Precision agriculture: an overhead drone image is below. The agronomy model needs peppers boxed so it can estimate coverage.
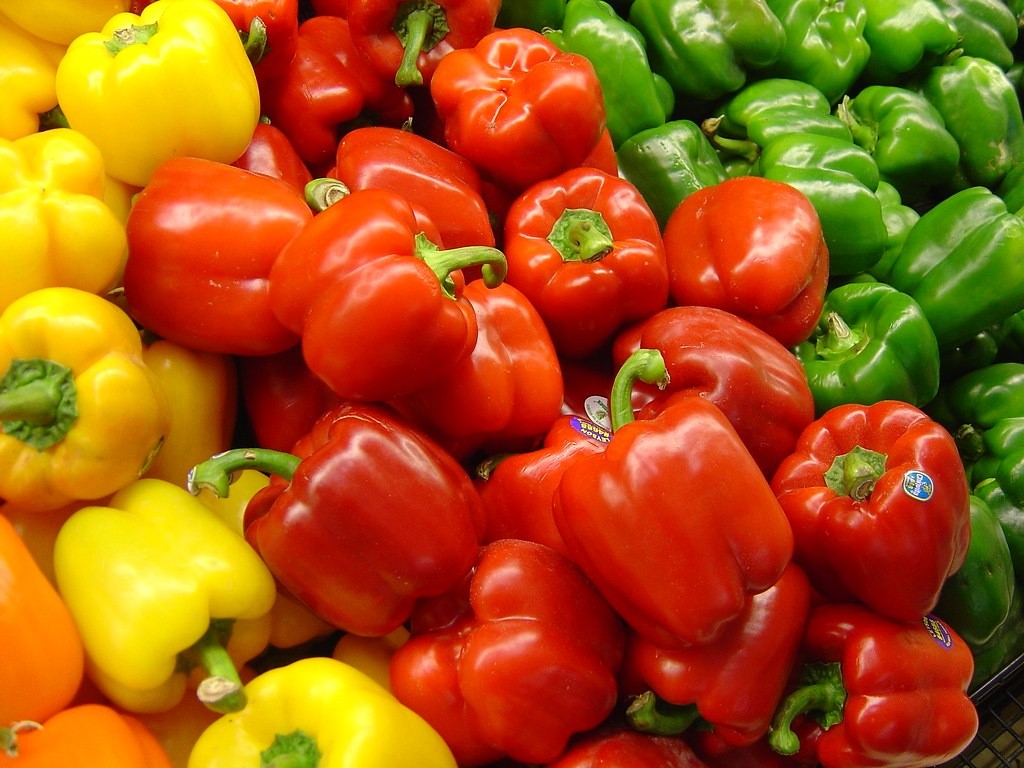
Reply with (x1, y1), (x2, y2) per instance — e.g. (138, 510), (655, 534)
(0, 0), (1024, 768)
